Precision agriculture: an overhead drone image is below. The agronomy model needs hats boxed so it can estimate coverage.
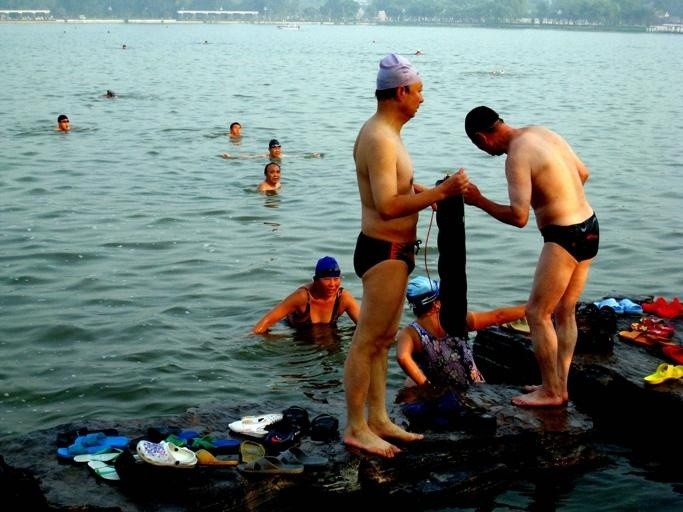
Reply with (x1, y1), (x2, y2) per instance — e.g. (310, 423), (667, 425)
(406, 276), (437, 307)
(376, 53), (422, 91)
(57, 115), (67, 122)
(315, 256), (340, 278)
(269, 139), (279, 148)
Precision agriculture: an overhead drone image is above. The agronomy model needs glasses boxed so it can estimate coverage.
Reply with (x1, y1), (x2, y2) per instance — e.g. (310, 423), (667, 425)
(316, 269), (339, 277)
(271, 145), (280, 148)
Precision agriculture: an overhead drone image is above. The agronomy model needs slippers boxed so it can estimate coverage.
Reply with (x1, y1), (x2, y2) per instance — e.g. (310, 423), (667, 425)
(498, 295), (682, 386)
(56, 404), (342, 481)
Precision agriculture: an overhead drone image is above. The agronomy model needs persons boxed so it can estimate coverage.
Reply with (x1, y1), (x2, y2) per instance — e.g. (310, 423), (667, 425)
(221, 139), (320, 160)
(255, 163), (280, 192)
(224, 122), (241, 140)
(340, 52), (471, 459)
(248, 253), (359, 335)
(54, 114), (70, 131)
(396, 276), (527, 387)
(461, 106), (600, 407)
(102, 90), (119, 99)
